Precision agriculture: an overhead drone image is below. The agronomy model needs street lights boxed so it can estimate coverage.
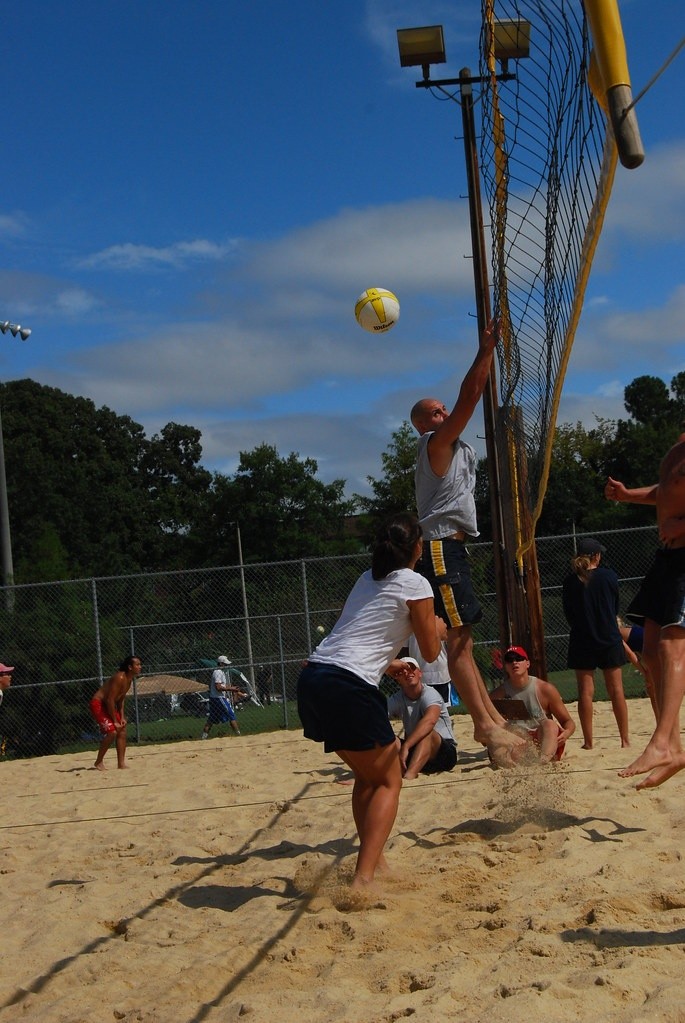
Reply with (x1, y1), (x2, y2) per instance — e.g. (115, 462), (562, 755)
(0, 315), (31, 614)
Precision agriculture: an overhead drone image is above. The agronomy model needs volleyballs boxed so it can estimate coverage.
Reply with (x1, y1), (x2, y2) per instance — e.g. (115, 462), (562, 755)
(354, 286), (400, 334)
(317, 625), (325, 634)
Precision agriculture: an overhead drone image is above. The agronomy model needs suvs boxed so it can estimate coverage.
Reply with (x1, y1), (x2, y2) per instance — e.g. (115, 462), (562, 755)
(132, 696), (156, 711)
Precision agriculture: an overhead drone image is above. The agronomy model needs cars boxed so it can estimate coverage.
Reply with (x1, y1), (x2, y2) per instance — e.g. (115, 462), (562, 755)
(165, 691), (231, 714)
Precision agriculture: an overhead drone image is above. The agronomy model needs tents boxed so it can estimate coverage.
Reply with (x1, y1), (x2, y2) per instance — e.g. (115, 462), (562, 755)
(126, 675), (210, 700)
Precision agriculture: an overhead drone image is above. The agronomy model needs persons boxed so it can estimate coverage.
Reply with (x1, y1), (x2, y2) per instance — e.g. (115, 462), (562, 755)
(87, 656), (142, 771)
(296, 517), (448, 894)
(489, 643), (503, 688)
(606, 419), (685, 793)
(563, 539), (657, 749)
(487, 647), (575, 769)
(410, 320), (527, 747)
(256, 666), (272, 705)
(338, 657), (457, 784)
(199, 656), (248, 739)
(0, 663), (14, 705)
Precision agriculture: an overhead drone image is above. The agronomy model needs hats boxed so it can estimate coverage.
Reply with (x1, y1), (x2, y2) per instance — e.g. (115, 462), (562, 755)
(0, 662), (15, 674)
(503, 645), (532, 663)
(215, 656), (231, 665)
(397, 657), (422, 671)
(577, 538), (608, 560)
